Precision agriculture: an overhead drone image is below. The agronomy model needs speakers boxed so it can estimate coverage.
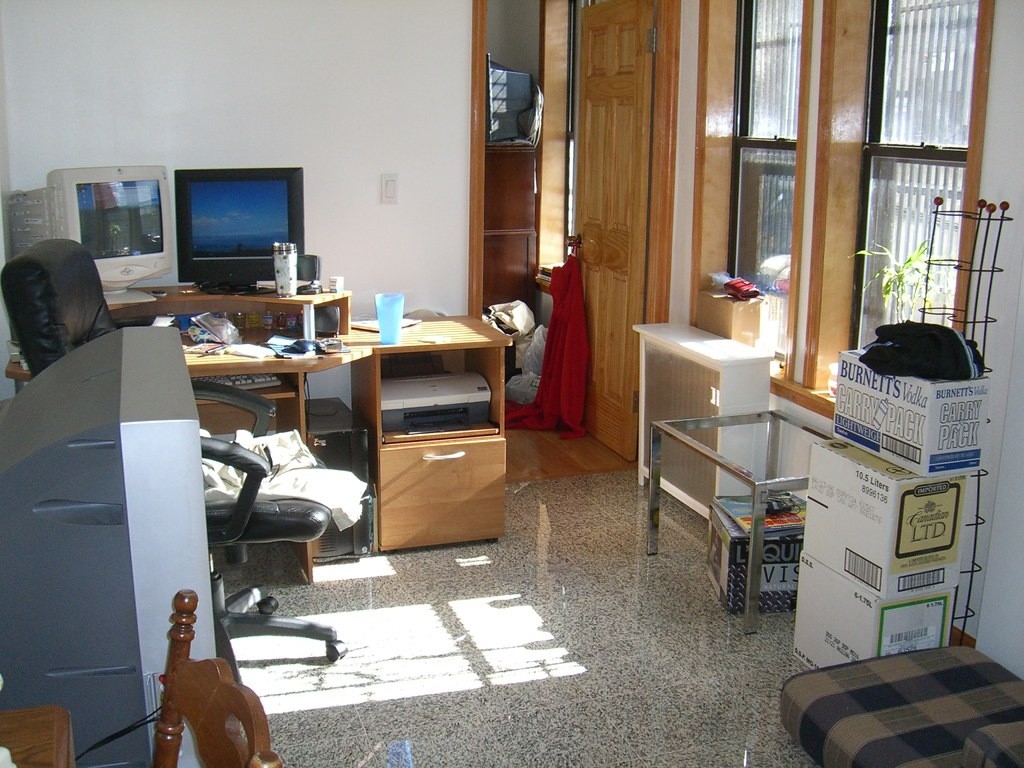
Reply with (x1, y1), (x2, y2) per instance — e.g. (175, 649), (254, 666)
(314, 305), (340, 337)
(296, 253), (322, 296)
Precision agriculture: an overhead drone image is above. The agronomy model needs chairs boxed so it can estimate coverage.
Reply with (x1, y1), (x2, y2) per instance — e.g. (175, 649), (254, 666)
(152, 587), (289, 768)
(0, 236), (351, 683)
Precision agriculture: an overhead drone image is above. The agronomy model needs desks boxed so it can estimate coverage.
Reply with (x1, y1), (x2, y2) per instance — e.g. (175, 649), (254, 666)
(0, 704), (75, 768)
(6, 285), (364, 564)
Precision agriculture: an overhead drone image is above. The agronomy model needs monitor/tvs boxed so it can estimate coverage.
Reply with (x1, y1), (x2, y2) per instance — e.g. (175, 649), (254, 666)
(173, 167), (304, 294)
(485, 52), (534, 144)
(7, 165), (174, 305)
(0, 325), (216, 768)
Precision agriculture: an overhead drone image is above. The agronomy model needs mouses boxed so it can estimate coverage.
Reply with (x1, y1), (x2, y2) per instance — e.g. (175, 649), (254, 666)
(289, 339), (314, 353)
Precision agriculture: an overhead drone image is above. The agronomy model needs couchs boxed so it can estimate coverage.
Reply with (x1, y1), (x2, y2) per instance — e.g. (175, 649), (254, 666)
(779, 645), (1024, 768)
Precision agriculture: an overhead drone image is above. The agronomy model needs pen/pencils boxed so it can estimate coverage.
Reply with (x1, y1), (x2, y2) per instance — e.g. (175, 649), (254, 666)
(205, 345), (227, 354)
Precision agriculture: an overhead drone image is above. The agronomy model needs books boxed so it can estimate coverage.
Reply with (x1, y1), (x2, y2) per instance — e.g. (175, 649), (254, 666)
(536, 262), (564, 286)
(713, 493), (807, 535)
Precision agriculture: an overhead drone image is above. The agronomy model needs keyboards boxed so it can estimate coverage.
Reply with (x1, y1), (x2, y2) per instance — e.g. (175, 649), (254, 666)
(192, 372), (281, 390)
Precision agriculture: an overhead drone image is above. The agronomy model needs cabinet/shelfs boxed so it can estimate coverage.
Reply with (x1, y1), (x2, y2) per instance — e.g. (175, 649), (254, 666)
(484, 137), (536, 320)
(353, 309), (516, 555)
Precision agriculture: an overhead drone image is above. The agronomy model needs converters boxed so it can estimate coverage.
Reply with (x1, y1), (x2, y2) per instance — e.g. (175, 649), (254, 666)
(768, 500), (795, 513)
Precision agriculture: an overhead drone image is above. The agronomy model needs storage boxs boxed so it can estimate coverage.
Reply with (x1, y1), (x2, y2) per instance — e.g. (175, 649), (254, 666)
(792, 553), (956, 673)
(697, 287), (766, 336)
(803, 438), (970, 600)
(702, 493), (808, 614)
(832, 345), (995, 478)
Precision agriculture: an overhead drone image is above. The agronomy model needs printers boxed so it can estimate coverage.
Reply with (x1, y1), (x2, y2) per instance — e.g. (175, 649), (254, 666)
(380, 371), (492, 434)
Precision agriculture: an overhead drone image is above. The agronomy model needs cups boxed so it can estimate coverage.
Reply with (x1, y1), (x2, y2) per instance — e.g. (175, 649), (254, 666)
(376, 293), (405, 345)
(274, 243), (298, 298)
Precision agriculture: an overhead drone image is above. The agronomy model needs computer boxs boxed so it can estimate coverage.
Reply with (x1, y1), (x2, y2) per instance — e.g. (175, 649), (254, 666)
(304, 397), (371, 563)
